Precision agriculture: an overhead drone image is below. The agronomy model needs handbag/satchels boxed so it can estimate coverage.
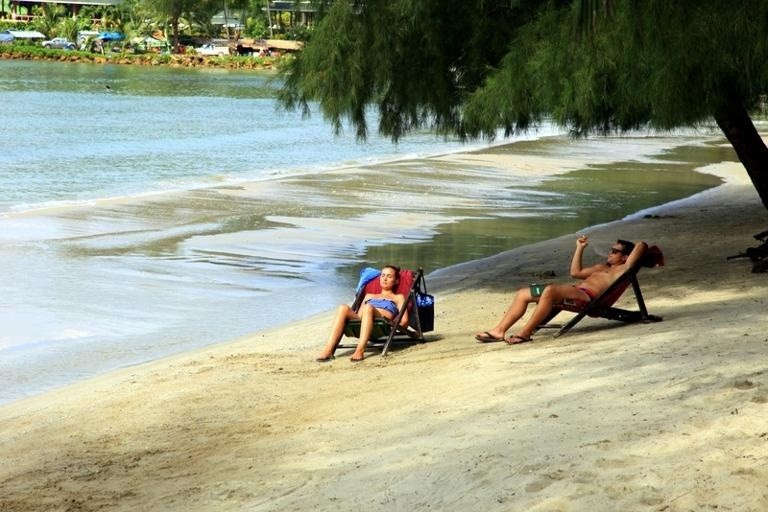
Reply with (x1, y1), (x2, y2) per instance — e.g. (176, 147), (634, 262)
(409, 291), (434, 332)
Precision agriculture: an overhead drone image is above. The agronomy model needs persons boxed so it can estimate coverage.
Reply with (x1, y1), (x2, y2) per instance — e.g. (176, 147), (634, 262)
(473, 233), (650, 344)
(316, 264), (409, 363)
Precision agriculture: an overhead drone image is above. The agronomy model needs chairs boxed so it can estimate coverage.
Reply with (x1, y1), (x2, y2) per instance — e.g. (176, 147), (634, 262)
(532, 247), (663, 340)
(336, 264), (427, 357)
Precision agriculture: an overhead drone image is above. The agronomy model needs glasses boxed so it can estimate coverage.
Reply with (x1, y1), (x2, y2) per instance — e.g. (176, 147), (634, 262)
(609, 247), (623, 254)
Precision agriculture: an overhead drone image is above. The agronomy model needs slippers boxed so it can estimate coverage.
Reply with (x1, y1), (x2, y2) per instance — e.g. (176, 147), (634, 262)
(505, 335), (532, 345)
(475, 332), (504, 342)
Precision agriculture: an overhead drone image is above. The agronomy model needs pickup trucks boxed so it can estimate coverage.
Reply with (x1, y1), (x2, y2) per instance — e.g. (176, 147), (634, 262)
(41, 37), (76, 50)
(195, 42), (229, 58)
